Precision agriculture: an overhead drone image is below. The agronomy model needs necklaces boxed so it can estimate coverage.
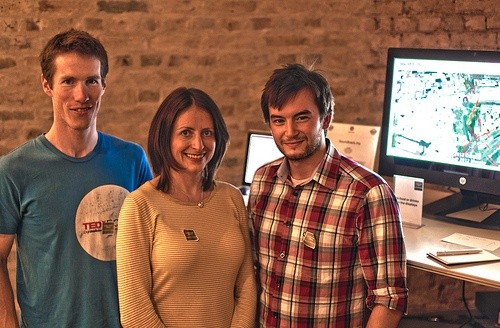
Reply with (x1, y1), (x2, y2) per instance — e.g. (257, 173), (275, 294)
(171, 181), (204, 208)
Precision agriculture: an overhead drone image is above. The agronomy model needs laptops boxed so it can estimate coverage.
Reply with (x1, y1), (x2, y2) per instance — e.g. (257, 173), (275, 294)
(238, 130), (284, 206)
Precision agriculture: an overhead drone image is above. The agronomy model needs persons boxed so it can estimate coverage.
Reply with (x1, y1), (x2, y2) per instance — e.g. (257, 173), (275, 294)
(246, 63), (409, 328)
(0, 28), (153, 328)
(115, 86), (258, 328)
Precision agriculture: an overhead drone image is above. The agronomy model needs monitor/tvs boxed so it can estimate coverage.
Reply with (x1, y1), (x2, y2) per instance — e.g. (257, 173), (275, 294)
(378, 47), (500, 230)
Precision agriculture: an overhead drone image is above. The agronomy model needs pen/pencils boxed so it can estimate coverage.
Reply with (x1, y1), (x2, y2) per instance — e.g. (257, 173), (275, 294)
(437, 248), (483, 256)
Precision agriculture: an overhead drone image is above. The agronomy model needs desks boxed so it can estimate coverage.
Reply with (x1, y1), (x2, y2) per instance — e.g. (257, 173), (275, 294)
(400, 189), (500, 290)
